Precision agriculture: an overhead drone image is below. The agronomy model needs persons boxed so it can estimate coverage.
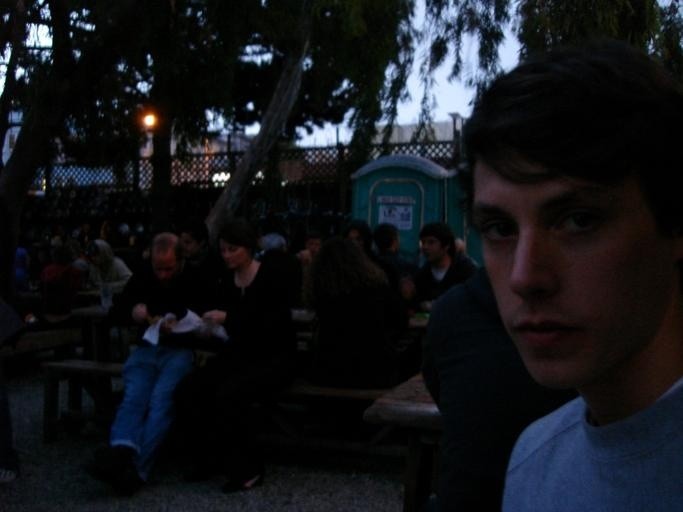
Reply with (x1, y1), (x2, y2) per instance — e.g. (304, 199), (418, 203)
(0, 38), (682, 511)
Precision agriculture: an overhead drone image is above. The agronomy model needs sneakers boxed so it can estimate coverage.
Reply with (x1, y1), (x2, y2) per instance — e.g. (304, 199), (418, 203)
(142, 464), (168, 486)
(0, 468), (18, 485)
(220, 473), (264, 494)
(109, 464), (140, 494)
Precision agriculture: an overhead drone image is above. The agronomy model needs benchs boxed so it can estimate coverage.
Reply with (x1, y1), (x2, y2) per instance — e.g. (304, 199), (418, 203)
(35, 355), (390, 461)
